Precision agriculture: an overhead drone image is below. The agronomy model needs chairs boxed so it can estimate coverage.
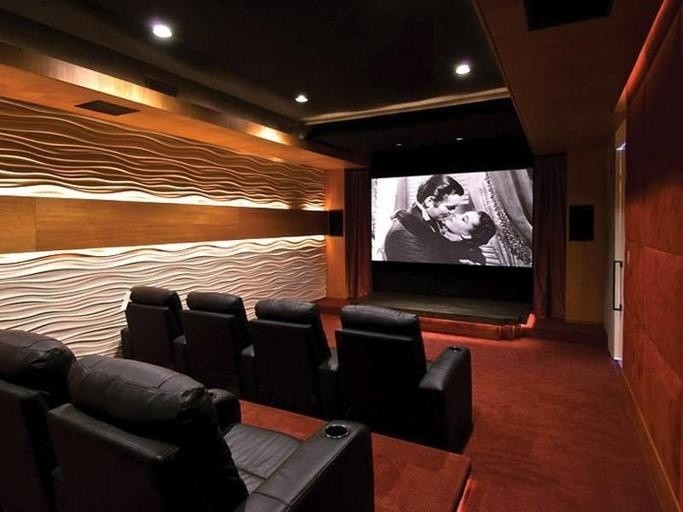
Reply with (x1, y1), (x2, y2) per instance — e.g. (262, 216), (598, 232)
(246, 298), (334, 408)
(178, 290), (254, 388)
(43, 352), (375, 512)
(120, 285), (182, 368)
(328, 305), (471, 444)
(1, 328), (76, 512)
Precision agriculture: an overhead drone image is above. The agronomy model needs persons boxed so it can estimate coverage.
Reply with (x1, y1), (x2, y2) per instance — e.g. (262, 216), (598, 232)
(391, 210), (496, 265)
(384, 176), (486, 265)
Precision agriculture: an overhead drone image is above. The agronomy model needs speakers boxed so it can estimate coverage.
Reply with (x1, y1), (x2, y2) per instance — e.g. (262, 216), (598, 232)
(567, 204), (595, 243)
(328, 209), (343, 237)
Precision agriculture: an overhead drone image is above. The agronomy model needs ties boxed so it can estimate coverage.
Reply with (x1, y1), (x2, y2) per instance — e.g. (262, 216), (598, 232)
(429, 219), (438, 233)
